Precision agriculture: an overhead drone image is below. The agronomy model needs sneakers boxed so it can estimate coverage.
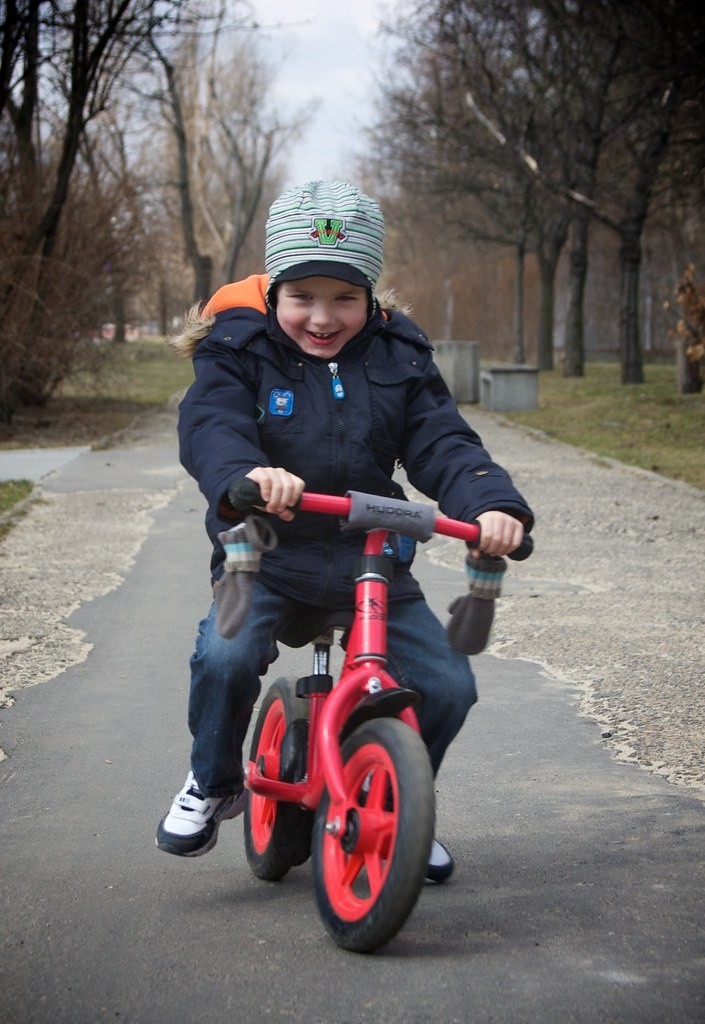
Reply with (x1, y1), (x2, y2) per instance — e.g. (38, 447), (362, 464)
(154, 768), (251, 861)
(364, 792), (454, 881)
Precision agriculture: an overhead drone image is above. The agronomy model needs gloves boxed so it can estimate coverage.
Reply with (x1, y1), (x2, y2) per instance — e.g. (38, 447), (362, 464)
(449, 554), (507, 657)
(214, 518), (265, 636)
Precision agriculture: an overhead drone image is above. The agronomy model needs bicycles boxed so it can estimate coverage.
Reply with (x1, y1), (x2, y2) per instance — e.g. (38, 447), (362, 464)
(226, 476), (535, 955)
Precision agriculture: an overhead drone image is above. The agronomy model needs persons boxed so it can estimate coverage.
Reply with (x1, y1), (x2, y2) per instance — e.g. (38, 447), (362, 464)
(156, 179), (535, 882)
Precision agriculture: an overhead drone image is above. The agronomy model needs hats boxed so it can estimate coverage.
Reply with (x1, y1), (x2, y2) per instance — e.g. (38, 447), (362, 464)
(262, 177), (386, 316)
